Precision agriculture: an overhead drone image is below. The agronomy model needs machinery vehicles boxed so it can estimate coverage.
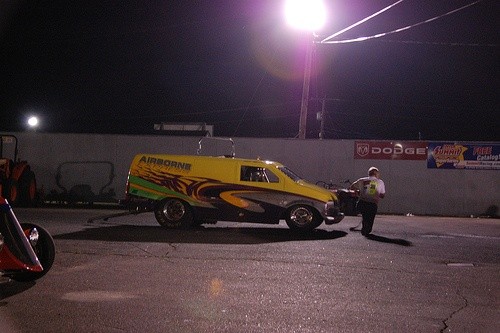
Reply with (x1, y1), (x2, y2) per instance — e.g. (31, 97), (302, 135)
(0, 134), (37, 207)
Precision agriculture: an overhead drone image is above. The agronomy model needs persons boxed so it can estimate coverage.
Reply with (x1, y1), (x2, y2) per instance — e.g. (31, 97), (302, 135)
(251, 173), (265, 182)
(350, 167), (385, 238)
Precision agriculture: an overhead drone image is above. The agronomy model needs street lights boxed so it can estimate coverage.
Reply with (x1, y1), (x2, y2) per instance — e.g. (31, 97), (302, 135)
(294, 0), (317, 140)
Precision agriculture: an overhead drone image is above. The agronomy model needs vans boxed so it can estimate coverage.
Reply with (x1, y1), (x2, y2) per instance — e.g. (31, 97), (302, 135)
(120, 153), (344, 231)
